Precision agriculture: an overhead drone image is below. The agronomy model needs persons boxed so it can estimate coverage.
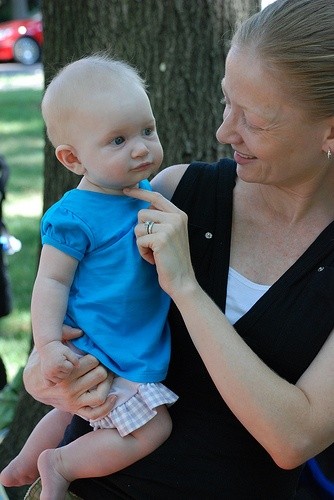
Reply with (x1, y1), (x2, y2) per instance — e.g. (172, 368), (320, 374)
(23, 0), (334, 499)
(0, 53), (179, 500)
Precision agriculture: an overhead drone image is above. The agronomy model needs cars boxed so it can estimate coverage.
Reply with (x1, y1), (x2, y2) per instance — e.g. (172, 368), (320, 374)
(0, 10), (43, 65)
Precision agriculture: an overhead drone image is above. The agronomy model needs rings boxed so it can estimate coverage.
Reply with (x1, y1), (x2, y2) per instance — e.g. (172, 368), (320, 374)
(145, 221), (153, 234)
